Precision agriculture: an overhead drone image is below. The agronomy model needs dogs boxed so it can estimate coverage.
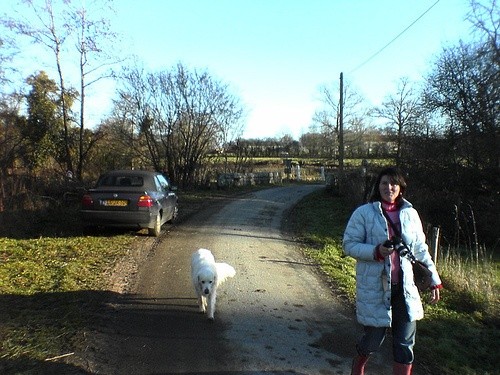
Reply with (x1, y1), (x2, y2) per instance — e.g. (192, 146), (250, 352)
(191, 246), (237, 318)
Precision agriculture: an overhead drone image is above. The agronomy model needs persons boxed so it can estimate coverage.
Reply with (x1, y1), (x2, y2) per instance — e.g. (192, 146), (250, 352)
(342, 167), (443, 375)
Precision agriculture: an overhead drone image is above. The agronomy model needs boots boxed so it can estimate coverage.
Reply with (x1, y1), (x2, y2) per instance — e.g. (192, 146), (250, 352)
(393, 362), (412, 375)
(351, 355), (368, 375)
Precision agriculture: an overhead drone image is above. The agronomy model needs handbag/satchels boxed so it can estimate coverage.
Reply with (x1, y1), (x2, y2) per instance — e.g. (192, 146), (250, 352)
(411, 259), (433, 291)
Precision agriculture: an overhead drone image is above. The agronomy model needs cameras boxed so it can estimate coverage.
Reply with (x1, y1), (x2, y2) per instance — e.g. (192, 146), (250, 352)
(383, 235), (408, 257)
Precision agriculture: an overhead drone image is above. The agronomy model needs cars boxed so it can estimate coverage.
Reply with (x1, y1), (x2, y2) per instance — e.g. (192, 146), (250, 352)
(78, 169), (179, 237)
